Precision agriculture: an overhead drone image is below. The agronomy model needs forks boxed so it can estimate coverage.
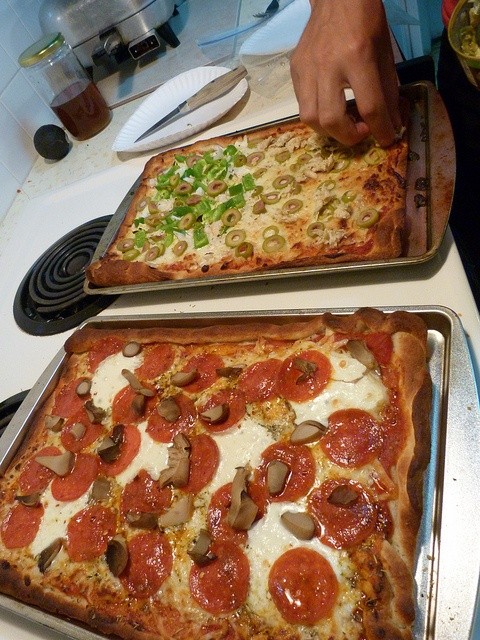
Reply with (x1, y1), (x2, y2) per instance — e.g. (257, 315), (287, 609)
(251, 0), (280, 19)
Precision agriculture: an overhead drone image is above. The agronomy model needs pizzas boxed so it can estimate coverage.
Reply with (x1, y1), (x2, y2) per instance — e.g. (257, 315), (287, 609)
(87, 105), (409, 287)
(1, 307), (432, 639)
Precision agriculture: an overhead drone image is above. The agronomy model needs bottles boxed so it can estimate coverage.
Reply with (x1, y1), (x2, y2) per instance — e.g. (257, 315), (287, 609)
(17, 31), (114, 142)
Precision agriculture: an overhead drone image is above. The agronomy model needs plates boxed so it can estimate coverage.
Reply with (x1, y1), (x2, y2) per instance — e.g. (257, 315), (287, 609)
(110, 65), (249, 152)
(237, 0), (312, 57)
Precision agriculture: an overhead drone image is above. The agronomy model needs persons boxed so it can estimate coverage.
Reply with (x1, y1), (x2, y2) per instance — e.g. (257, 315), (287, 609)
(290, 1), (402, 147)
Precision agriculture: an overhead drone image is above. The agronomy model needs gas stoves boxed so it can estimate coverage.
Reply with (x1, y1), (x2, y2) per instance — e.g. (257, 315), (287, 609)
(0, 93), (479, 640)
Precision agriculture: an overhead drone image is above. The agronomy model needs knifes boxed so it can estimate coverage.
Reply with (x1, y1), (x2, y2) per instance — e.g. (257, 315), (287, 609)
(133, 65), (248, 143)
